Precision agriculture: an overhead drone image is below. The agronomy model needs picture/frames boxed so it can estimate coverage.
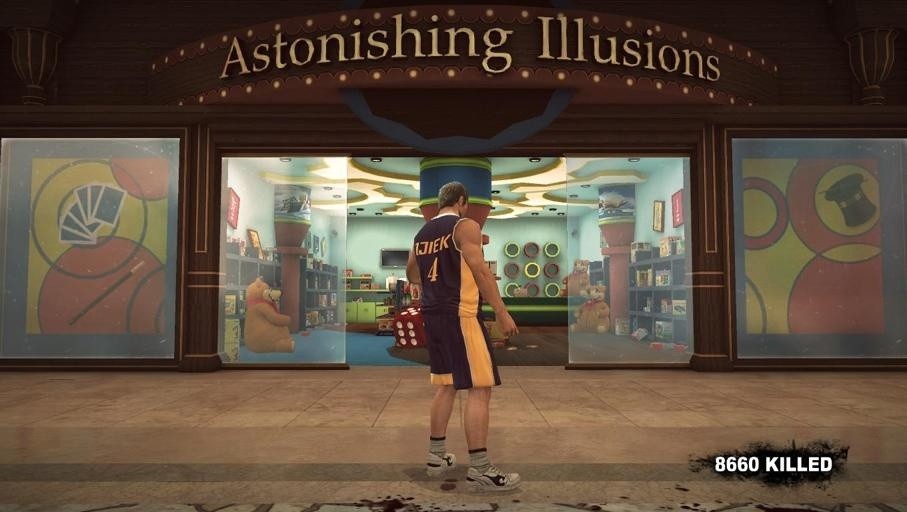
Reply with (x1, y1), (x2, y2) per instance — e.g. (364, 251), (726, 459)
(653, 200), (665, 232)
(672, 188), (684, 228)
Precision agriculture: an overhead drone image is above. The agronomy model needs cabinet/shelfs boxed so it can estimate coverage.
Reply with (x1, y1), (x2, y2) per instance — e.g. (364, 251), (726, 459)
(588, 246), (689, 349)
(224, 246), (412, 346)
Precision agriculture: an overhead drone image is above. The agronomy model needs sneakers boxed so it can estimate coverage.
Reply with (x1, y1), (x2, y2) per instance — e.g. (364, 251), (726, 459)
(465, 466), (521, 492)
(427, 453), (456, 478)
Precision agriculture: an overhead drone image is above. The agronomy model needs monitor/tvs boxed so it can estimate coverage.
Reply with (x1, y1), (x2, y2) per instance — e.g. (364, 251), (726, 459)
(381, 248), (413, 268)
